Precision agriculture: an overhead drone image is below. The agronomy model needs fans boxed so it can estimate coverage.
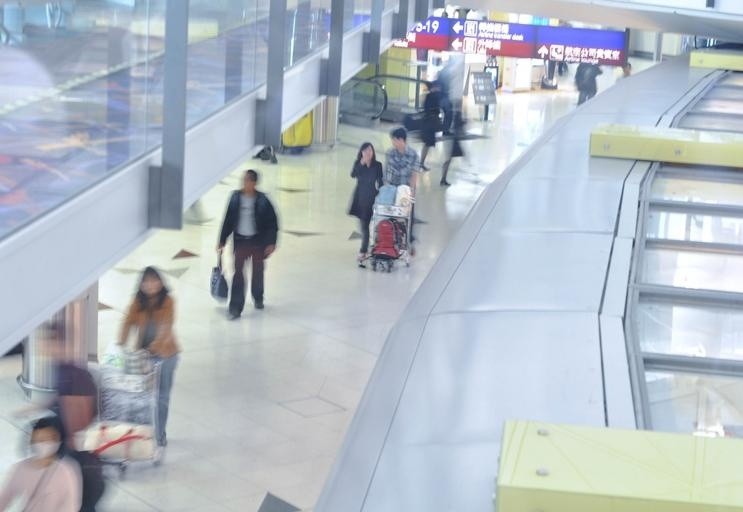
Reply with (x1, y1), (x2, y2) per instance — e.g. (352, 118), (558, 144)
(472, 72), (497, 105)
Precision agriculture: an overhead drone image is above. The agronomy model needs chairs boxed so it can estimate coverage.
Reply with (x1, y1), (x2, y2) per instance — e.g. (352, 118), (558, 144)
(225, 309), (241, 320)
(356, 246), (370, 269)
(419, 162), (431, 171)
(254, 296), (265, 309)
(439, 179), (452, 186)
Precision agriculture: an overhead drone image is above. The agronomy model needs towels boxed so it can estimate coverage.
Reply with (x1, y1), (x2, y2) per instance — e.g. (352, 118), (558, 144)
(16, 292), (90, 397)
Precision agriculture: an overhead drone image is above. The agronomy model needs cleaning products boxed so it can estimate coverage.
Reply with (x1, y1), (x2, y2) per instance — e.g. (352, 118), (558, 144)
(93, 350), (164, 481)
(359, 183), (412, 273)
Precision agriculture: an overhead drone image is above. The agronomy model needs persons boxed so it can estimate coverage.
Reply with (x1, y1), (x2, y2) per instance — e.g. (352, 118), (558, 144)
(35, 321), (107, 511)
(345, 142), (382, 262)
(439, 97), (464, 186)
(116, 263), (178, 467)
(0, 418), (85, 512)
(420, 79), (452, 171)
(216, 170), (278, 320)
(615, 62), (631, 80)
(574, 62), (590, 106)
(383, 127), (421, 246)
(582, 64), (601, 100)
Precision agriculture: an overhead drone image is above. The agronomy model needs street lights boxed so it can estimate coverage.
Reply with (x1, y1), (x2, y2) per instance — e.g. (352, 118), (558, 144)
(209, 250), (229, 304)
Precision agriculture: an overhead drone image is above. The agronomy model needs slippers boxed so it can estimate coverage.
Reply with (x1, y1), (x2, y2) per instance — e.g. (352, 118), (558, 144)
(370, 216), (407, 260)
